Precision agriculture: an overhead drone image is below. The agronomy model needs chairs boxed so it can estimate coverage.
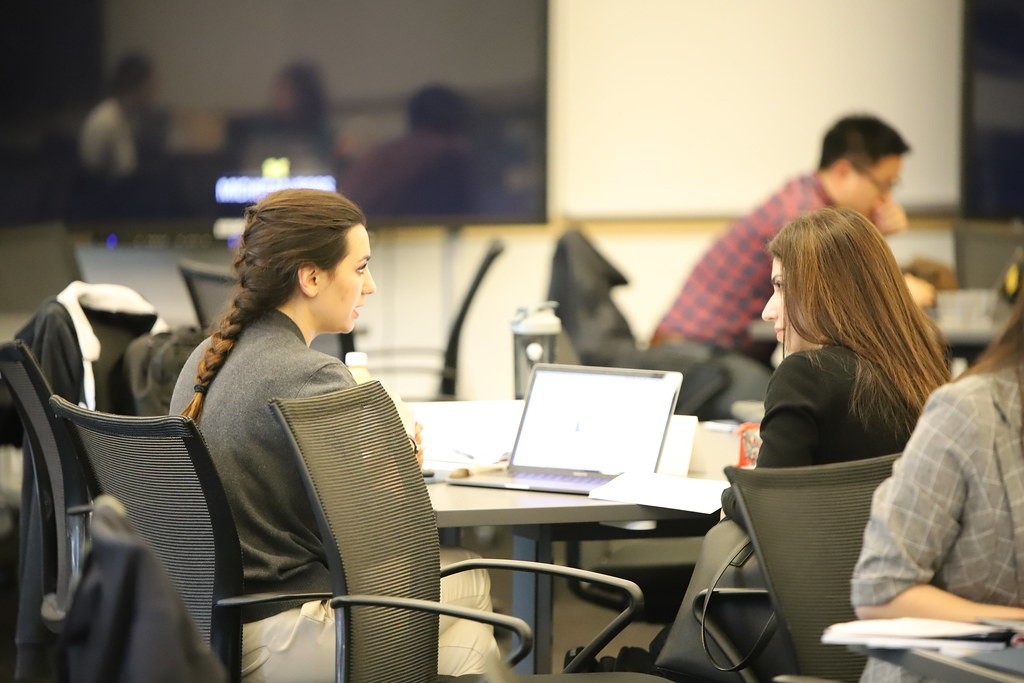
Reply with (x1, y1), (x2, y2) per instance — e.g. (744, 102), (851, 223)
(0, 219), (1024, 683)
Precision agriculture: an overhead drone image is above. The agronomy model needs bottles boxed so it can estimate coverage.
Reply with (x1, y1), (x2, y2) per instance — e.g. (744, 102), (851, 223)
(341, 349), (375, 389)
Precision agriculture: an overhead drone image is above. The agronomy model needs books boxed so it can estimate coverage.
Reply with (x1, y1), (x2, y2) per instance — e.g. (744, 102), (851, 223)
(822, 616), (1015, 650)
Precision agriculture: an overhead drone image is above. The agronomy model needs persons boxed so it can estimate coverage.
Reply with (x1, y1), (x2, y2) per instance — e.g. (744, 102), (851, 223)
(718, 207), (951, 534)
(171, 188), (500, 683)
(81, 56), (176, 179)
(652, 118), (936, 360)
(851, 289), (1024, 683)
(341, 83), (511, 214)
(235, 66), (358, 166)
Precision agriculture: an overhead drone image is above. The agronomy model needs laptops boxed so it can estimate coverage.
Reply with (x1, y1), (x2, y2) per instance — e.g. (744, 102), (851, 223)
(446, 363), (684, 496)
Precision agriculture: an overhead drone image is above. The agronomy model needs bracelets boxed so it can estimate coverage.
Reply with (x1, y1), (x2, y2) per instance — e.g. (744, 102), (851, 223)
(408, 435), (418, 454)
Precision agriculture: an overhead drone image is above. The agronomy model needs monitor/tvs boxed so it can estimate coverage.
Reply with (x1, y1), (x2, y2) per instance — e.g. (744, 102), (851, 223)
(0, 0), (549, 244)
(957, 0), (1024, 224)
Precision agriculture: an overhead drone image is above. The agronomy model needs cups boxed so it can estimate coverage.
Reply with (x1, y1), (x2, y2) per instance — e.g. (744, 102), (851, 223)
(512, 312), (561, 401)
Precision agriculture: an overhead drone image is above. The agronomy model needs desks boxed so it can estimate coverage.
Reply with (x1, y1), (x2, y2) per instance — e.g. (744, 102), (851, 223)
(398, 401), (762, 675)
(747, 284), (1009, 373)
(845, 619), (1024, 683)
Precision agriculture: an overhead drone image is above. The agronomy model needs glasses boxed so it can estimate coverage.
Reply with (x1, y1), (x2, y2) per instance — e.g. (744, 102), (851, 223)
(851, 162), (894, 200)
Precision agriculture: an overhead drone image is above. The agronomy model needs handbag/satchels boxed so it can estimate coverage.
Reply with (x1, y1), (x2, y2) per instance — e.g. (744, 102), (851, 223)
(652, 520), (800, 683)
